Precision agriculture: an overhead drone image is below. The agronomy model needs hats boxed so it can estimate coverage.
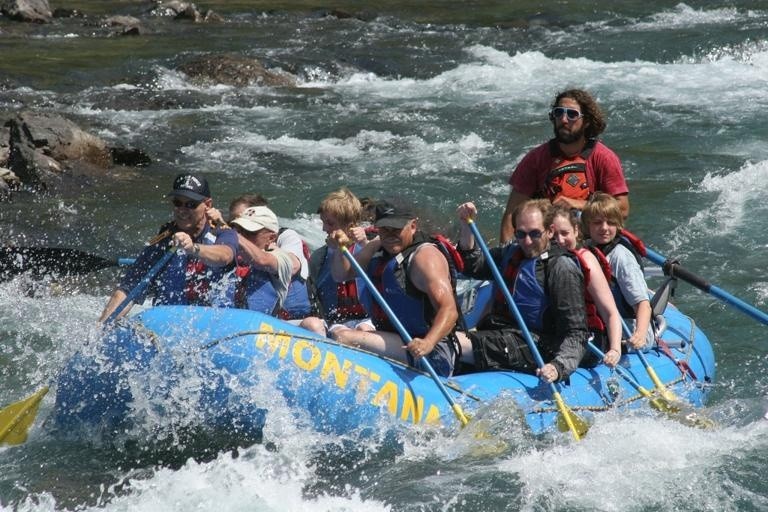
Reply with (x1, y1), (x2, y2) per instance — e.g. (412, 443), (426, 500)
(169, 175), (210, 200)
(374, 197), (414, 229)
(230, 205), (279, 234)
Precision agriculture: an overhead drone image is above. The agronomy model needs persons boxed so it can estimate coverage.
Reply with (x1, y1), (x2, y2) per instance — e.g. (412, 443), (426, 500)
(498, 87), (630, 246)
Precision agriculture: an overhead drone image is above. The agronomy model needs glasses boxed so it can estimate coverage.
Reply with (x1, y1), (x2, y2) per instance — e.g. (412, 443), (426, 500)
(173, 198), (208, 209)
(553, 107), (584, 121)
(514, 228), (547, 238)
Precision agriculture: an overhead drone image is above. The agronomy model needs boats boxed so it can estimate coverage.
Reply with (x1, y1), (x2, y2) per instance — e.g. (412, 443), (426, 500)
(44, 281), (720, 466)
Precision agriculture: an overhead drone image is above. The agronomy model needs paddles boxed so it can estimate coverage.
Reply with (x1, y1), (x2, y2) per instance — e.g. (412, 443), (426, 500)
(336, 241), (506, 454)
(0, 240), (177, 446)
(467, 213), (588, 440)
(584, 306), (678, 416)
